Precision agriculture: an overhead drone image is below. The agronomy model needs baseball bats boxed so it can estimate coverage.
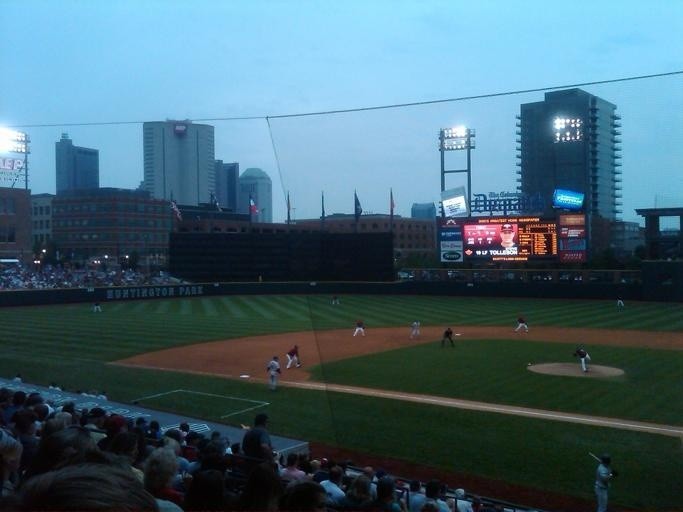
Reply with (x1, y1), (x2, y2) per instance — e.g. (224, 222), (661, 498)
(589, 452), (613, 471)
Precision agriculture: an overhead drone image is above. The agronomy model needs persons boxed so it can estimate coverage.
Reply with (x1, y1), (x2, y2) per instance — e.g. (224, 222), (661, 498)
(495, 224), (517, 250)
(352, 319), (366, 337)
(331, 294), (340, 305)
(93, 298), (103, 313)
(264, 355), (283, 392)
(0, 262), (179, 289)
(13, 373), (24, 383)
(616, 295), (625, 312)
(410, 319), (421, 340)
(441, 326), (456, 348)
(594, 452), (619, 512)
(573, 343), (591, 372)
(514, 314), (528, 333)
(286, 344), (303, 370)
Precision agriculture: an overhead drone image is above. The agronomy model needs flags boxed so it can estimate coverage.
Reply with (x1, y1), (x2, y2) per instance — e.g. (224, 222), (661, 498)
(249, 195), (259, 215)
(355, 194), (362, 221)
(213, 193), (223, 212)
(391, 194), (395, 209)
(172, 200), (184, 222)
